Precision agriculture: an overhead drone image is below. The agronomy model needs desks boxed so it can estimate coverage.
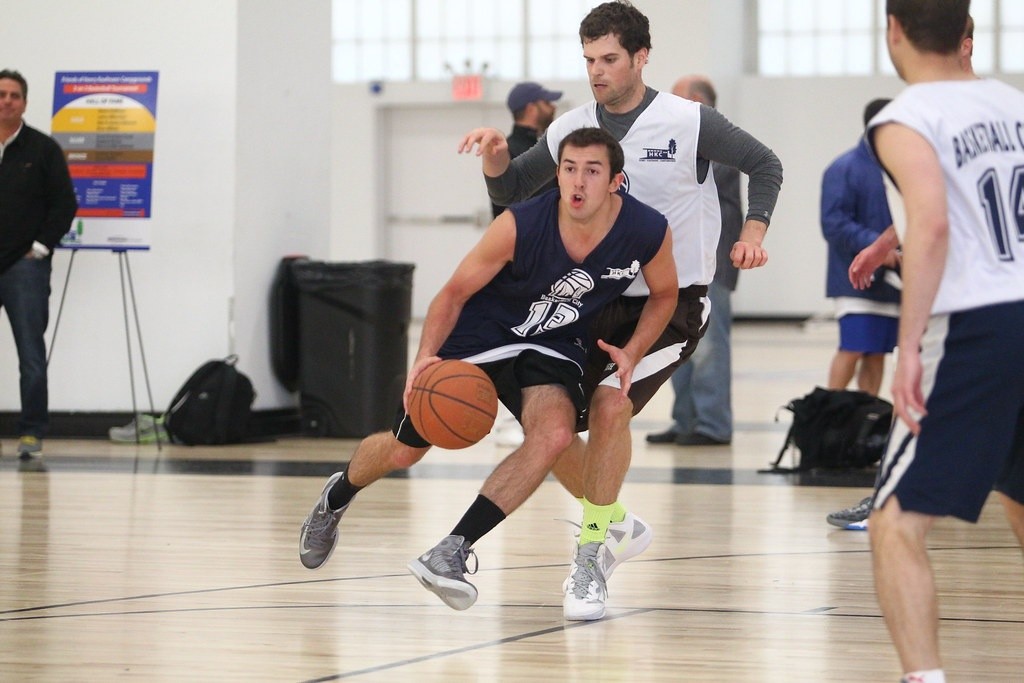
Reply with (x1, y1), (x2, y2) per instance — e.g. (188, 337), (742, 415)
(47, 245), (165, 452)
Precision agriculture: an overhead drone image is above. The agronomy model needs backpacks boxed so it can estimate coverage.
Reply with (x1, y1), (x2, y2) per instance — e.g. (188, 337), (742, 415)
(163, 355), (254, 445)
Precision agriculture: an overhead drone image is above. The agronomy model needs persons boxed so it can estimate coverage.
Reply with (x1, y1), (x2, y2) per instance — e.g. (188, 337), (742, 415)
(299, 125), (679, 615)
(825, 14), (979, 530)
(646, 74), (743, 446)
(491, 83), (562, 221)
(0, 69), (76, 459)
(821, 99), (903, 397)
(848, 0), (1024, 683)
(457, 2), (783, 620)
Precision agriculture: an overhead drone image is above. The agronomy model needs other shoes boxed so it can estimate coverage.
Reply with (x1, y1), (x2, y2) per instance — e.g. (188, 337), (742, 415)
(646, 430), (728, 444)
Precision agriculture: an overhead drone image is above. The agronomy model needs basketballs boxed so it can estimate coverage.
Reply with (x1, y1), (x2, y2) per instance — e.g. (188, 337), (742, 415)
(407, 357), (499, 450)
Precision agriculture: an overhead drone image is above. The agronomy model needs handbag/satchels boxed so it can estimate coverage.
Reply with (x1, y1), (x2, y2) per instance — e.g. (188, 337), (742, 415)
(762, 386), (894, 479)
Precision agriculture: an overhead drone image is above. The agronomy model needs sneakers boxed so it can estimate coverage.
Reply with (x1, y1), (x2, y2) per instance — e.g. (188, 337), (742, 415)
(16, 435), (44, 461)
(107, 414), (169, 443)
(298, 472), (357, 571)
(825, 496), (873, 531)
(407, 535), (479, 610)
(553, 507), (653, 594)
(563, 540), (610, 622)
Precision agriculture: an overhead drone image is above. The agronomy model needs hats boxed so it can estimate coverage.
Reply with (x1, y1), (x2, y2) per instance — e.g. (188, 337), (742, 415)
(506, 82), (563, 112)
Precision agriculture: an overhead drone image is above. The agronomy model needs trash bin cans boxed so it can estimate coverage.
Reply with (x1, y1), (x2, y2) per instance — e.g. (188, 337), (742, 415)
(269, 256), (420, 440)
